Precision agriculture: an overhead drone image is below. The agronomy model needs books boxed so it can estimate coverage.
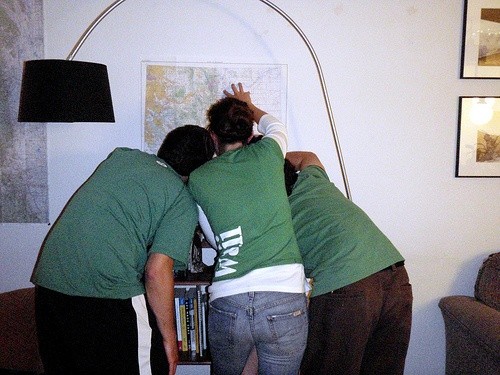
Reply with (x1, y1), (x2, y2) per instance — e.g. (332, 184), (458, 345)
(173, 285), (208, 360)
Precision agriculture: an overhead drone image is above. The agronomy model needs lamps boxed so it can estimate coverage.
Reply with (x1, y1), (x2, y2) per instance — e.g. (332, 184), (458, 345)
(18, 0), (352, 201)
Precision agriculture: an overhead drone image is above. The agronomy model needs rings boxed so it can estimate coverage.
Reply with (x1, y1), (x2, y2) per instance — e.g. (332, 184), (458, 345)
(235, 92), (239, 95)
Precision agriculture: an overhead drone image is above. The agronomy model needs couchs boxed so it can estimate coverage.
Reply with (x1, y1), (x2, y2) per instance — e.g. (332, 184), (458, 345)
(438, 252), (500, 375)
(0, 287), (45, 375)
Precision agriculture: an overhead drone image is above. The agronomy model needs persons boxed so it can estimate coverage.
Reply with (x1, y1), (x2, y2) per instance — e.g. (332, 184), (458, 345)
(185, 82), (309, 374)
(284, 151), (413, 375)
(30, 125), (214, 375)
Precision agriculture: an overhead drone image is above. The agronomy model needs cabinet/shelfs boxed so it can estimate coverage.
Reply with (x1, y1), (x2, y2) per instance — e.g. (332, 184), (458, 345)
(174, 225), (211, 365)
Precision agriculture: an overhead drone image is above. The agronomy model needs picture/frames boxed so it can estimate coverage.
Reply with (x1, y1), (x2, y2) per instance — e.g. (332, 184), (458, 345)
(455, 95), (500, 178)
(459, 0), (500, 80)
